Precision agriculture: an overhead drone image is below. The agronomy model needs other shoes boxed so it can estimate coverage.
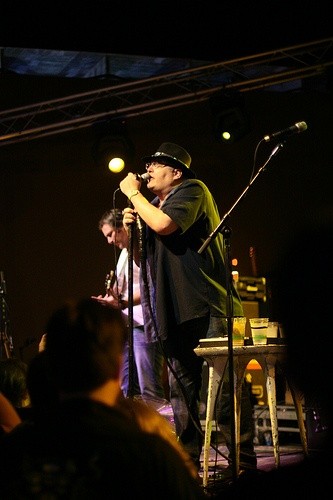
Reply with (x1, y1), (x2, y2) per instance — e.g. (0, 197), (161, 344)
(221, 464), (247, 479)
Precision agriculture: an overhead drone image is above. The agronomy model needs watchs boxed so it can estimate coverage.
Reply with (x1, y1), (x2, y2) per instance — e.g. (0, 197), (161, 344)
(128, 188), (140, 200)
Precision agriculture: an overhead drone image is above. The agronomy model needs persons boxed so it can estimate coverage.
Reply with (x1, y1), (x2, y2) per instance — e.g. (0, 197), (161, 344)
(91, 204), (160, 400)
(208, 245), (333, 500)
(0, 297), (207, 500)
(118, 143), (259, 479)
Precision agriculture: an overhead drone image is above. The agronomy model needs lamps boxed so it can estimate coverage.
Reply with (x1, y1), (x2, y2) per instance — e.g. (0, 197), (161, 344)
(209, 89), (247, 142)
(91, 120), (133, 175)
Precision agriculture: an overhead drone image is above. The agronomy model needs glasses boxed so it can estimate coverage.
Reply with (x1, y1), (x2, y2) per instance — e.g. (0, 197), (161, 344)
(145, 162), (168, 170)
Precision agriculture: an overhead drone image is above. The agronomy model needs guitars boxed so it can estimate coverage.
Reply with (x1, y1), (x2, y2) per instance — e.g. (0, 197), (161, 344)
(103, 271), (118, 301)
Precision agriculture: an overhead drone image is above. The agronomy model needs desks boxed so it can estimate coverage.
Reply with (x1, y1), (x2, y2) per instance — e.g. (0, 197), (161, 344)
(196, 344), (309, 487)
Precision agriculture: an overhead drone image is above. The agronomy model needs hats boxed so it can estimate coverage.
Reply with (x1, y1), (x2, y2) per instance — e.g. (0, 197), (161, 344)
(152, 143), (192, 173)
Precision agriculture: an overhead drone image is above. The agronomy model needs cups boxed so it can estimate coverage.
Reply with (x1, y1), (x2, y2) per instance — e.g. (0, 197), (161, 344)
(267, 322), (278, 339)
(227, 317), (246, 346)
(249, 318), (268, 345)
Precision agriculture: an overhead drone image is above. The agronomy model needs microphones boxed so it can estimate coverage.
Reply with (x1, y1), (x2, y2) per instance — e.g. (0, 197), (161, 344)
(118, 173), (150, 190)
(263, 121), (308, 142)
(107, 270), (114, 290)
